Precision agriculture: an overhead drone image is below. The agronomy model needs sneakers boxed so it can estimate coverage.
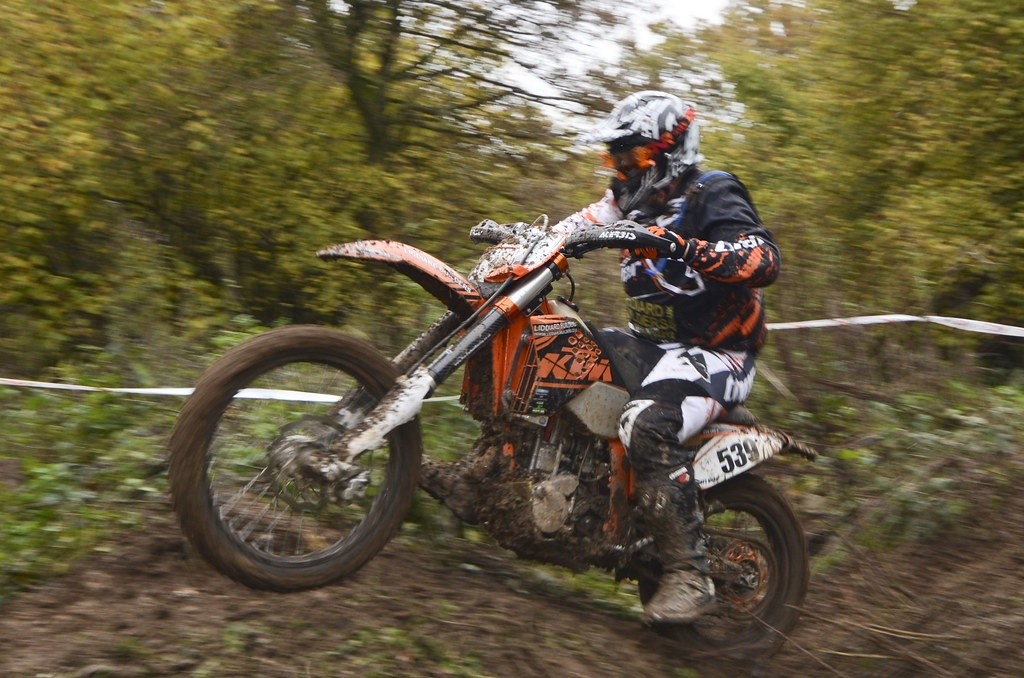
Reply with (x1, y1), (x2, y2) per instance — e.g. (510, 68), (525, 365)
(646, 567), (717, 622)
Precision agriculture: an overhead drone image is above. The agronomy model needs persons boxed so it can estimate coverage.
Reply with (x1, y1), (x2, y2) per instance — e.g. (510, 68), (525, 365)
(469, 88), (782, 626)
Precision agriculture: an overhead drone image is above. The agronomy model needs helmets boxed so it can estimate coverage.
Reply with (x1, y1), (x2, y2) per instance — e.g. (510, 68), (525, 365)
(598, 90), (700, 191)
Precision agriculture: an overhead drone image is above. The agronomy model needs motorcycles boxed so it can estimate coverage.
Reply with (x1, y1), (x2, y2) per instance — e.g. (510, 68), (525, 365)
(162, 212), (807, 671)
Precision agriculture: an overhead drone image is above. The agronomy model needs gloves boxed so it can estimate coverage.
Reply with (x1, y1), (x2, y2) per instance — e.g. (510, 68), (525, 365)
(630, 223), (687, 262)
